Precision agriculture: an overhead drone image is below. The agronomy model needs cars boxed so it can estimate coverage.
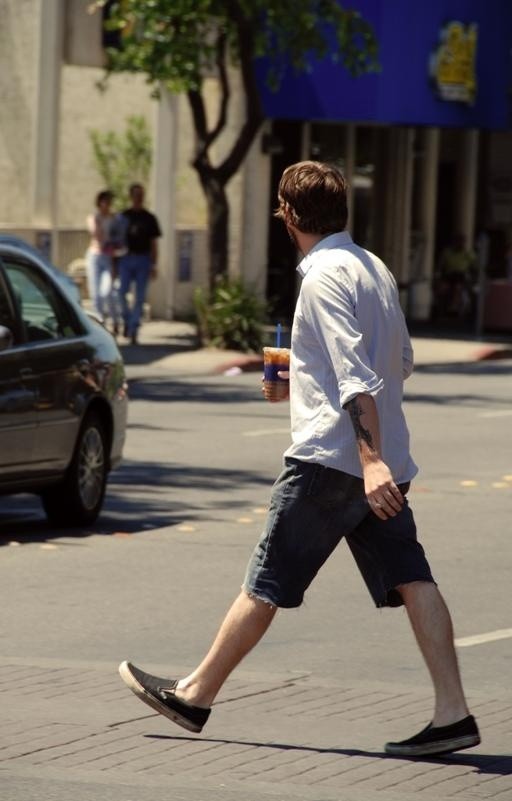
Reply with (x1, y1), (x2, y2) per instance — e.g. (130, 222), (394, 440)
(1, 231), (130, 525)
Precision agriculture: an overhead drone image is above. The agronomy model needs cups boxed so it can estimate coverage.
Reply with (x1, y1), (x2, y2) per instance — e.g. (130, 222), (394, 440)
(261, 347), (291, 404)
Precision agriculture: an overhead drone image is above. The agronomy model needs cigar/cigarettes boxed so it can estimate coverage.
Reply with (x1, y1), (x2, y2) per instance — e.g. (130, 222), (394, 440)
(374, 501), (382, 508)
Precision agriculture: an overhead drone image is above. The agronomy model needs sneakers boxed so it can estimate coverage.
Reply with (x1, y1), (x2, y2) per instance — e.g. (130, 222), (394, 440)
(385, 715), (480, 756)
(120, 659), (212, 734)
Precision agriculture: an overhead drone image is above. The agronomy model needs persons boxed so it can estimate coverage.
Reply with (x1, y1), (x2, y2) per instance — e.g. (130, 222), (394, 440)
(120, 159), (479, 760)
(108, 183), (162, 347)
(87, 189), (119, 337)
